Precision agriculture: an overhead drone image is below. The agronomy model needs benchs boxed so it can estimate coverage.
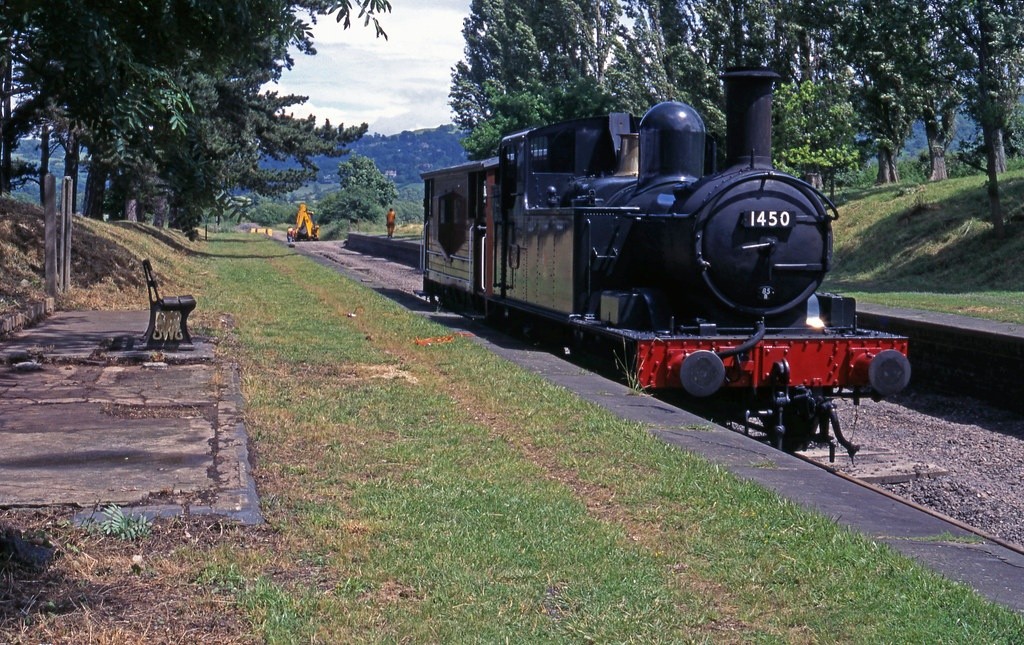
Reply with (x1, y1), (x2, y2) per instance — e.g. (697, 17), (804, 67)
(142, 259), (196, 347)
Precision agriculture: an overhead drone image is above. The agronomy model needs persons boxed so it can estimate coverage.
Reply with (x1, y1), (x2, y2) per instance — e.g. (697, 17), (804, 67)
(287, 226), (296, 242)
(385, 207), (395, 238)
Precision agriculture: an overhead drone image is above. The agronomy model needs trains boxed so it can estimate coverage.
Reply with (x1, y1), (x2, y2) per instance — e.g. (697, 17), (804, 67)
(411, 71), (912, 456)
